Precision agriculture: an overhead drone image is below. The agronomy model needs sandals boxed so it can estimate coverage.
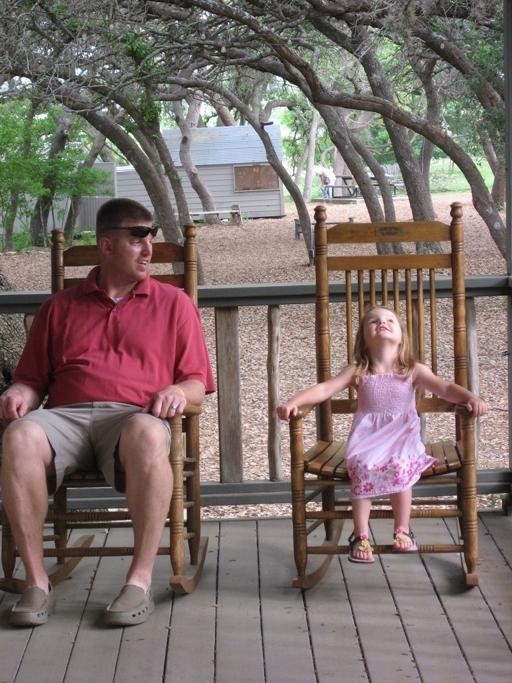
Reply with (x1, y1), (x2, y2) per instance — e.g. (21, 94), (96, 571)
(394, 526), (418, 552)
(348, 531), (375, 563)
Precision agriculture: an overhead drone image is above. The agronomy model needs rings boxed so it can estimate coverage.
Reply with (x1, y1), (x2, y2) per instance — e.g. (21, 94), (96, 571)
(170, 405), (177, 409)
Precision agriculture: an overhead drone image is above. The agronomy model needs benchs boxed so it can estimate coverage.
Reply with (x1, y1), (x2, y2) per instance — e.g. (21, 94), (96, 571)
(174, 204), (243, 224)
(373, 183), (404, 195)
(294, 218), (354, 240)
(323, 184), (359, 198)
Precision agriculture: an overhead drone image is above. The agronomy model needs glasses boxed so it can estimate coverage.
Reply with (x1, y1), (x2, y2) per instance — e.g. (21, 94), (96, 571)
(103, 226), (159, 238)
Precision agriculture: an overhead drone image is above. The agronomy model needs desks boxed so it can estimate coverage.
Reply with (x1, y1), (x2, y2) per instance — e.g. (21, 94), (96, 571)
(335, 175), (358, 196)
(371, 175), (393, 180)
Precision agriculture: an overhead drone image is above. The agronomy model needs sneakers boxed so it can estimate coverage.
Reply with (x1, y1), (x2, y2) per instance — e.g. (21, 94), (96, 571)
(11, 583), (56, 627)
(105, 584), (156, 624)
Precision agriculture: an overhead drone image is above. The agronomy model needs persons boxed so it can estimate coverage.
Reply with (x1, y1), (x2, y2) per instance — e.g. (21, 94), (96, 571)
(274, 304), (490, 565)
(0, 194), (217, 636)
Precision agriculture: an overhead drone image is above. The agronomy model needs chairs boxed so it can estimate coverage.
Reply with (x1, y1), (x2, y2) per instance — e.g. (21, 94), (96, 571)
(0, 222), (209, 598)
(289, 202), (479, 589)
